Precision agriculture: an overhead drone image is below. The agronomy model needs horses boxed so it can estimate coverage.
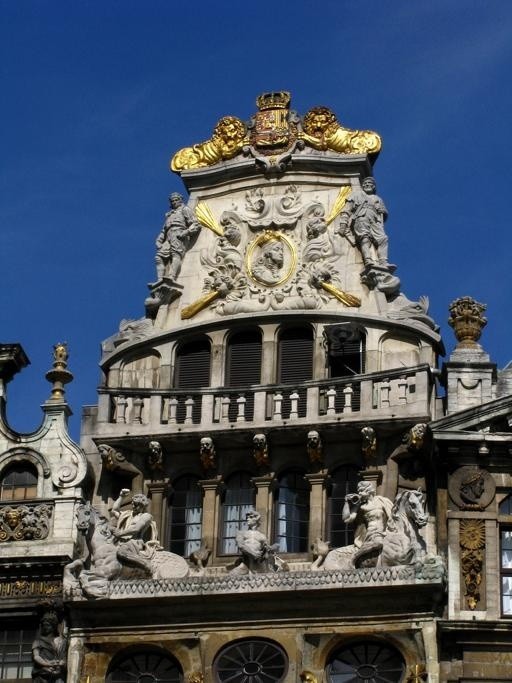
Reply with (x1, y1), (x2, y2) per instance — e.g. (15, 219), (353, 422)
(63, 501), (214, 598)
(309, 486), (437, 573)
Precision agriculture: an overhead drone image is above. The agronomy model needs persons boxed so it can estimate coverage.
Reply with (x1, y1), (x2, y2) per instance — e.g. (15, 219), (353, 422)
(98, 444), (111, 458)
(228, 510), (267, 575)
(460, 473), (485, 504)
(6, 507), (22, 530)
(146, 192), (201, 290)
(111, 488), (164, 559)
(31, 611), (68, 683)
(341, 479), (395, 568)
(252, 238), (286, 284)
(414, 422), (427, 440)
(337, 176), (397, 276)
